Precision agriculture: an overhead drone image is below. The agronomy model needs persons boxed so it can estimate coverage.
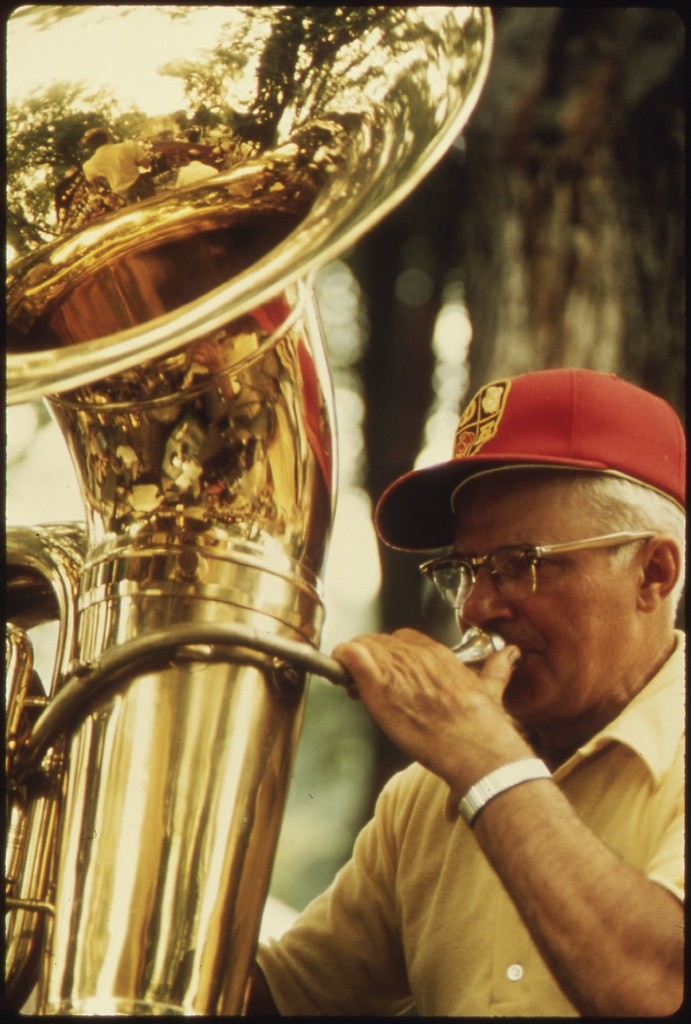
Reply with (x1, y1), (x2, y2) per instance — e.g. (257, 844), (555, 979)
(246, 367), (687, 1018)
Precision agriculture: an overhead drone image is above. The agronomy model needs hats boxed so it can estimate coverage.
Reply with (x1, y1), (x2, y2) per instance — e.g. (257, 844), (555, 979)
(375, 368), (687, 552)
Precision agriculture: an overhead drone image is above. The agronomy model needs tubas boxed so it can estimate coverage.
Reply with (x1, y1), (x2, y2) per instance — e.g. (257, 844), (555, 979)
(1, 0), (496, 1022)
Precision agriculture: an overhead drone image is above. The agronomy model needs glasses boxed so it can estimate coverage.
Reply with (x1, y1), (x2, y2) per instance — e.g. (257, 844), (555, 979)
(418, 531), (657, 609)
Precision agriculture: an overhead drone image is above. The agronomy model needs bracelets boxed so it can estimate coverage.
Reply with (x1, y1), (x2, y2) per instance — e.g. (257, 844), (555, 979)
(458, 757), (554, 825)
